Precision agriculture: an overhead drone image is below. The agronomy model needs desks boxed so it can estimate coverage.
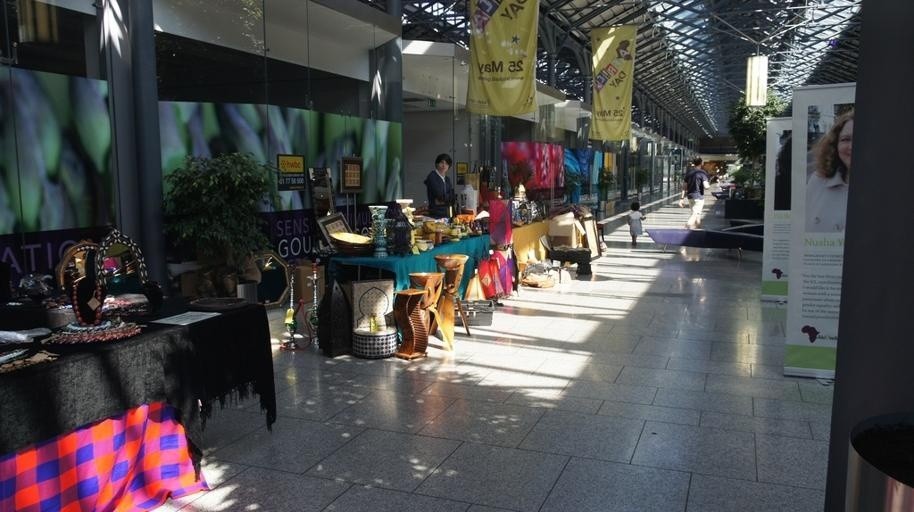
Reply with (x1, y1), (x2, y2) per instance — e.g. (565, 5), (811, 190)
(0, 295), (276, 512)
(324, 203), (607, 344)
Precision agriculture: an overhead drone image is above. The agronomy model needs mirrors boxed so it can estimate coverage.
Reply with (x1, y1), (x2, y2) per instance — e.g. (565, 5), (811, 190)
(245, 250), (291, 310)
(94, 227), (148, 290)
(55, 239), (98, 291)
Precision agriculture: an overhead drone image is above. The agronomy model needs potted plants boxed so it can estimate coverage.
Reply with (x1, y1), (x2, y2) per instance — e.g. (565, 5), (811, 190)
(597, 166), (616, 211)
(634, 168), (651, 201)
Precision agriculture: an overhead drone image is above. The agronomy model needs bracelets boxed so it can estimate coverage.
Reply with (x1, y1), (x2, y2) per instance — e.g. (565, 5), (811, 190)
(679, 198), (684, 199)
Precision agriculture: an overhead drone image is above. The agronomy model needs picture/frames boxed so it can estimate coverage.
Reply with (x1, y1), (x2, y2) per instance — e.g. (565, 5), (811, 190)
(340, 155), (365, 194)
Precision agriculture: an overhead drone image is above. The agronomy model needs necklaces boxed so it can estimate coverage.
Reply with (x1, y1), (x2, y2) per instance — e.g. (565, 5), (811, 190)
(73, 278), (102, 327)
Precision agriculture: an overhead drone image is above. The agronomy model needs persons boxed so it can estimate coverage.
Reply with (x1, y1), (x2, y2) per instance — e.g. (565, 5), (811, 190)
(627, 202), (646, 246)
(679, 158), (710, 228)
(424, 154), (455, 220)
(807, 110), (855, 232)
